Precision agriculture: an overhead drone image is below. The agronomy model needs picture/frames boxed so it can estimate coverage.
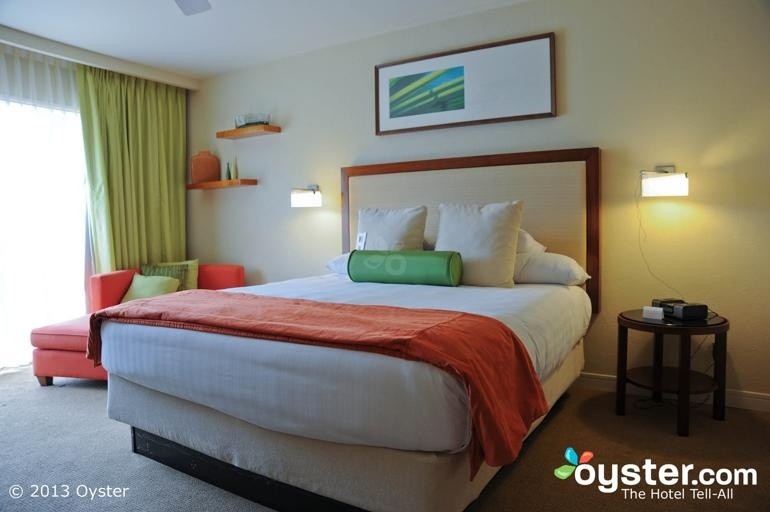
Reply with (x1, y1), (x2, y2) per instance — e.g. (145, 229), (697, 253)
(375, 32), (556, 137)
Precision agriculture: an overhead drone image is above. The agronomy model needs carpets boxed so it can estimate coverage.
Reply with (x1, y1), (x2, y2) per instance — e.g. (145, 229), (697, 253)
(1, 365), (281, 511)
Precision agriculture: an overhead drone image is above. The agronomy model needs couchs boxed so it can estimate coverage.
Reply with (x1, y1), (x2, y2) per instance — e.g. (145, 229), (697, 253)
(29, 264), (245, 388)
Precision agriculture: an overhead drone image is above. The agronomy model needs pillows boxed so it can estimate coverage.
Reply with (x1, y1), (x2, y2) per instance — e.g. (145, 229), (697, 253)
(347, 248), (463, 287)
(157, 258), (199, 290)
(141, 263), (187, 293)
(435, 200), (523, 289)
(325, 252), (350, 274)
(512, 252), (590, 287)
(422, 225), (546, 253)
(120, 271), (180, 305)
(355, 203), (428, 253)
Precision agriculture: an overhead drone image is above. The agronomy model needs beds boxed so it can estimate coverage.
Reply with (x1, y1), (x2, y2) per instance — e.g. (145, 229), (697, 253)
(87, 147), (598, 512)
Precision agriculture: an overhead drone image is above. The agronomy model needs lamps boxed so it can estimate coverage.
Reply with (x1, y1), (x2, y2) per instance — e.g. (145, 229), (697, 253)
(641, 171), (689, 198)
(290, 190), (322, 208)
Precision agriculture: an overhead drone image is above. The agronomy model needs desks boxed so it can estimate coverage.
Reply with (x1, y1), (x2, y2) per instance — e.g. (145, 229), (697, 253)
(617, 308), (730, 437)
